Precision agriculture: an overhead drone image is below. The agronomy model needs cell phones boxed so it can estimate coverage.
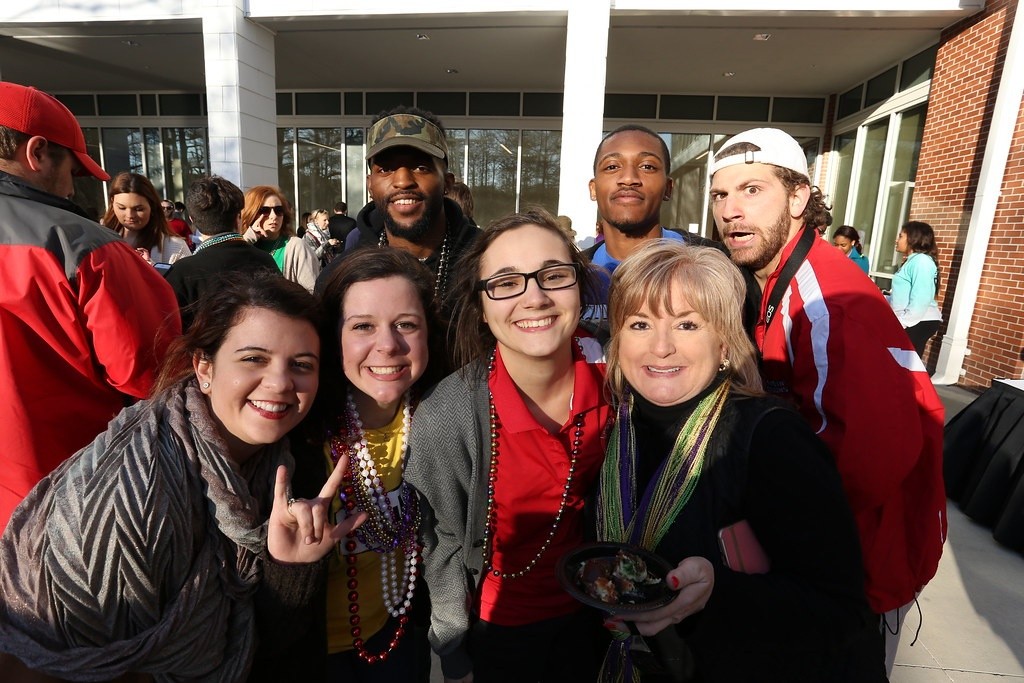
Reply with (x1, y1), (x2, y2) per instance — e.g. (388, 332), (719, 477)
(153, 262), (173, 278)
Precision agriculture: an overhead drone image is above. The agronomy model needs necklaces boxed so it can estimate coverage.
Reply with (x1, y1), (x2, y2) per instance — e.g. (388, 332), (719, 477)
(378, 226), (452, 310)
(482, 335), (587, 577)
(193, 233), (245, 258)
(328, 387), (435, 662)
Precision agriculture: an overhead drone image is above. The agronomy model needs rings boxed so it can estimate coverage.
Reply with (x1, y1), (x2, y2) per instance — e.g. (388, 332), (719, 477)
(289, 499), (311, 517)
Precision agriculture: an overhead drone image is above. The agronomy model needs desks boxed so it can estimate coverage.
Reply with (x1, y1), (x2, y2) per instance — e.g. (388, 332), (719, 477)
(940, 377), (1024, 554)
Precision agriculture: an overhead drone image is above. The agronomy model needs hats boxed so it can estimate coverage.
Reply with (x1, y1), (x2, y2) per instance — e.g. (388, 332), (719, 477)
(0, 81), (112, 181)
(364, 114), (449, 168)
(708, 128), (811, 184)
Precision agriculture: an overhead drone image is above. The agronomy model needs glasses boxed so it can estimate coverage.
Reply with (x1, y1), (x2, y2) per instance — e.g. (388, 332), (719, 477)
(818, 226), (826, 237)
(163, 206), (174, 211)
(259, 205), (287, 217)
(474, 263), (581, 300)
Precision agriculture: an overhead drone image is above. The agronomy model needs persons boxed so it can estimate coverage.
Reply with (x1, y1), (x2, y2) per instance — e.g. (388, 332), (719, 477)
(0, 265), (333, 683)
(101, 170), (193, 276)
(888, 222), (943, 360)
(710, 127), (947, 659)
(572, 223), (889, 683)
(403, 197), (622, 683)
(832, 226), (870, 276)
(297, 200), (356, 267)
(257, 245), (454, 683)
(578, 125), (780, 393)
(444, 182), (477, 226)
(0, 81), (193, 541)
(313, 106), (496, 400)
(159, 199), (197, 253)
(240, 185), (324, 295)
(163, 173), (284, 336)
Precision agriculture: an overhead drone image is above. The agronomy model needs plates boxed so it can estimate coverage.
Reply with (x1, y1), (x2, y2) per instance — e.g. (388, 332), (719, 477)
(562, 542), (680, 612)
